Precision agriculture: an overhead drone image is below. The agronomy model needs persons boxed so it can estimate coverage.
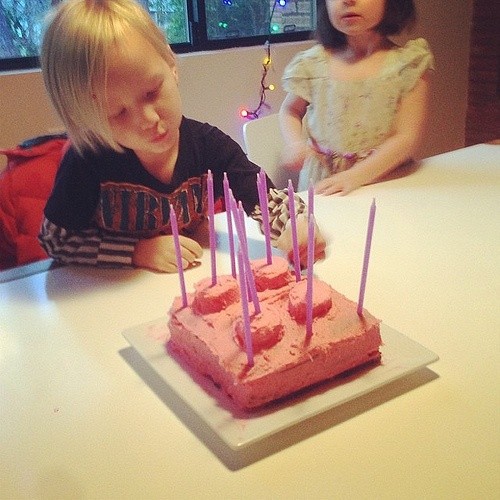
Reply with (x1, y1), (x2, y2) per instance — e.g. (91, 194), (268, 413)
(279, 0), (435, 197)
(37, 0), (327, 273)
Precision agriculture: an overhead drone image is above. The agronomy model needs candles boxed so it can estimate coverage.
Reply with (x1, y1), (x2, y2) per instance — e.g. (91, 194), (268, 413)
(168, 167), (378, 366)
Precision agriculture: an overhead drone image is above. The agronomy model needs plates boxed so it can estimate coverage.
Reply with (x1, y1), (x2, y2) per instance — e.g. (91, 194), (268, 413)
(121, 314), (439, 451)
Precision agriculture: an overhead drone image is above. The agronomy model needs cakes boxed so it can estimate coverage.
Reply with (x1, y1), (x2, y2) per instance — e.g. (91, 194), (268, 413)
(168, 256), (383, 411)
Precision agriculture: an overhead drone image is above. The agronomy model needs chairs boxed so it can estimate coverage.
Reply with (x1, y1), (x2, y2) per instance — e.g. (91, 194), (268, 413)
(243, 112), (302, 192)
(0, 136), (74, 270)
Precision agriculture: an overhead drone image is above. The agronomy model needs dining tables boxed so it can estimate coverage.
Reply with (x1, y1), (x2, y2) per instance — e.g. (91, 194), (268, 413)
(0, 139), (500, 498)
